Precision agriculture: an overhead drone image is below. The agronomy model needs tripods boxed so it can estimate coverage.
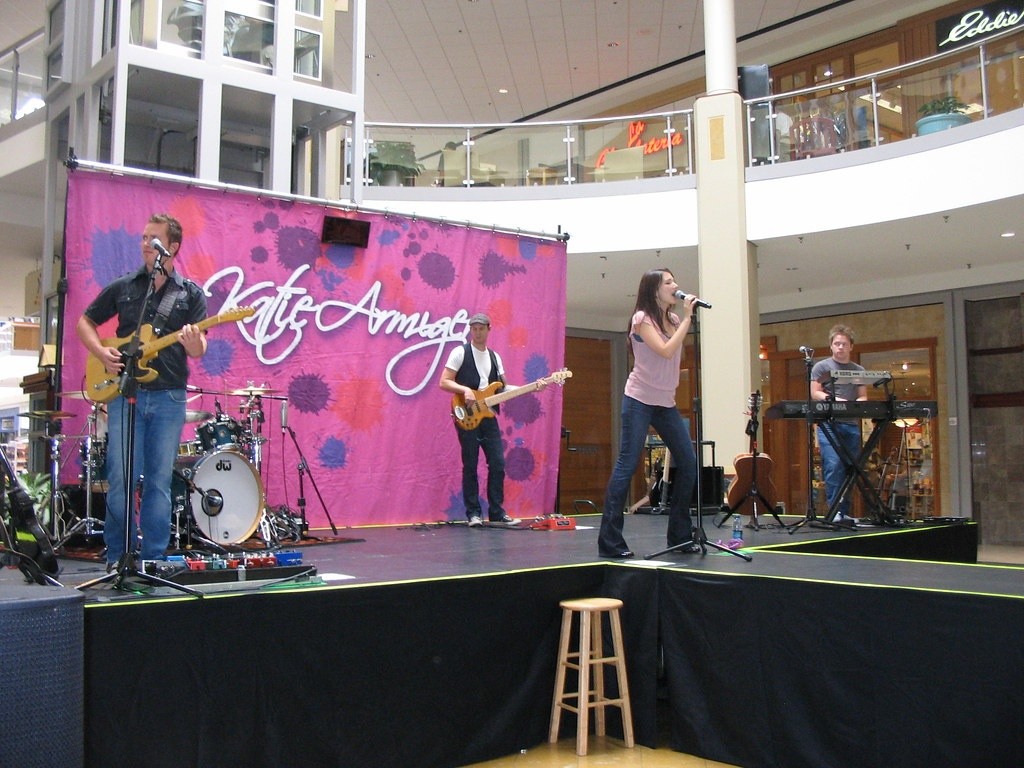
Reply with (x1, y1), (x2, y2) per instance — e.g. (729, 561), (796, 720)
(718, 389), (858, 531)
(35, 400), (211, 597)
(644, 308), (751, 560)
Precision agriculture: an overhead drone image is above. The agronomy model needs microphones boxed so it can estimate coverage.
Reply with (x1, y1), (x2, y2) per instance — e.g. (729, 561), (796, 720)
(186, 384), (202, 390)
(674, 290), (712, 309)
(280, 399), (289, 435)
(200, 488), (222, 507)
(150, 239), (171, 258)
(245, 383), (267, 407)
(799, 346), (813, 353)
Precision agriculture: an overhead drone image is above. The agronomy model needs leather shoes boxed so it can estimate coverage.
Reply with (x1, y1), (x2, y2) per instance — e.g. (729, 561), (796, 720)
(684, 547), (700, 553)
(620, 551), (633, 558)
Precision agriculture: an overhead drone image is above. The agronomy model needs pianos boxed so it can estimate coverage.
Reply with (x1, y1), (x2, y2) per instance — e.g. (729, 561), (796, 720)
(764, 401), (938, 529)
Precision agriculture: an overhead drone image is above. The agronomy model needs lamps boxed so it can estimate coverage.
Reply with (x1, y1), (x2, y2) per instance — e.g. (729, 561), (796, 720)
(891, 372), (918, 427)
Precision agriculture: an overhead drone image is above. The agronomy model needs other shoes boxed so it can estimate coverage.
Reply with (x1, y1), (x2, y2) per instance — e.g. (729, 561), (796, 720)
(832, 511), (860, 523)
(106, 562), (118, 574)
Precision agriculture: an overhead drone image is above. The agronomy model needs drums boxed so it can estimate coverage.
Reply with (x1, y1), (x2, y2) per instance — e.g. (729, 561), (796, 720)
(172, 449), (263, 547)
(197, 415), (238, 451)
(79, 438), (108, 481)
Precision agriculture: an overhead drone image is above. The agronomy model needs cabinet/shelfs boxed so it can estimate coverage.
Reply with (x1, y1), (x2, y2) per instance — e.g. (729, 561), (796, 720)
(814, 438), (938, 519)
(6, 440), (29, 476)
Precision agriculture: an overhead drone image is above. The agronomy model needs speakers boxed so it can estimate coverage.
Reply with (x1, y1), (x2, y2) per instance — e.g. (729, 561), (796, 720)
(322, 216), (371, 249)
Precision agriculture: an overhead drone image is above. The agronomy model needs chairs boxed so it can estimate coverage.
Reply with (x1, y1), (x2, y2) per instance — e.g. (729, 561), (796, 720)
(789, 116), (837, 160)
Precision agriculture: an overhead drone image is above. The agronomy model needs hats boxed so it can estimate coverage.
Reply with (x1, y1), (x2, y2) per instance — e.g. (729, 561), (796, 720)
(469, 313), (489, 325)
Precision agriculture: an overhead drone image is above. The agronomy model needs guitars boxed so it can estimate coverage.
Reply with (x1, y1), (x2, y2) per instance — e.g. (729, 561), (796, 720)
(727, 390), (779, 515)
(86, 305), (255, 404)
(450, 368), (573, 431)
(0, 449), (59, 584)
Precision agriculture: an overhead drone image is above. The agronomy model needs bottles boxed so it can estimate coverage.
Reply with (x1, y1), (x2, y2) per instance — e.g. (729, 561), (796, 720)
(732, 516), (743, 539)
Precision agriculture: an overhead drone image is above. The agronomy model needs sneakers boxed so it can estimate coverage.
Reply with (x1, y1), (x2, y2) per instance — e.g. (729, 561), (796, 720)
(468, 517), (481, 527)
(490, 515), (522, 526)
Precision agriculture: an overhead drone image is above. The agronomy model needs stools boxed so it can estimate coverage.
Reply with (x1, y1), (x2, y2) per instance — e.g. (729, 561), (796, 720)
(547, 596), (634, 756)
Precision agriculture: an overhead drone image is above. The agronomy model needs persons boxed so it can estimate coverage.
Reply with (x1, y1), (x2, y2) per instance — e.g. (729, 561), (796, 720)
(440, 313), (547, 526)
(809, 324), (866, 523)
(75, 214), (209, 573)
(880, 446), (908, 502)
(598, 268), (702, 558)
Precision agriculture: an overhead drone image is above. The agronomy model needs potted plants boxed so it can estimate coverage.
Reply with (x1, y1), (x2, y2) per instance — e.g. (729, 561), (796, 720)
(370, 141), (426, 186)
(916, 96), (972, 137)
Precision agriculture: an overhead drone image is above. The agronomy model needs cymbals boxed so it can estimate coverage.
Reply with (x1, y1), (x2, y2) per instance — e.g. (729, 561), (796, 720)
(17, 410), (76, 420)
(57, 391), (89, 400)
(240, 388), (282, 392)
(225, 386), (265, 395)
(185, 409), (212, 422)
